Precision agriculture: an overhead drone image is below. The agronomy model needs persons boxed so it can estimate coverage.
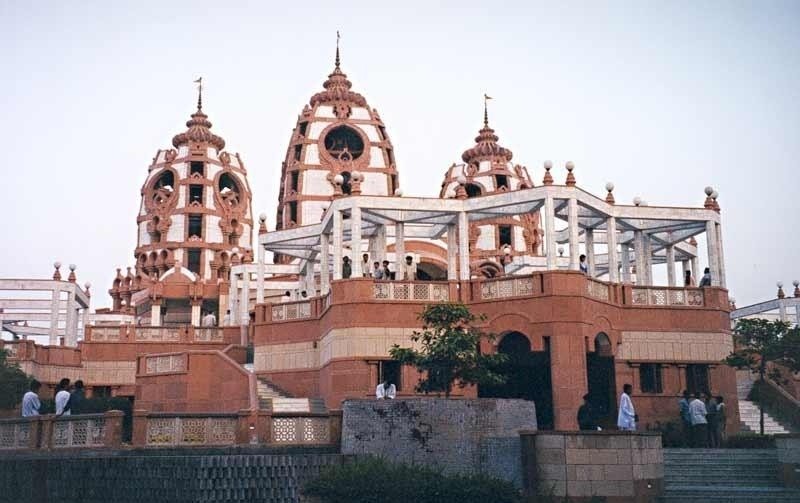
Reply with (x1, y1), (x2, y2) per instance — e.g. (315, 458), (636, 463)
(699, 268), (710, 287)
(617, 382), (639, 432)
(684, 270), (696, 287)
(577, 392), (603, 431)
(21, 379), (48, 448)
(678, 388), (726, 448)
(54, 378), (73, 445)
(400, 255), (419, 281)
(55, 379), (86, 444)
(342, 256), (351, 278)
(376, 377), (397, 400)
(580, 255), (587, 273)
(362, 253), (391, 281)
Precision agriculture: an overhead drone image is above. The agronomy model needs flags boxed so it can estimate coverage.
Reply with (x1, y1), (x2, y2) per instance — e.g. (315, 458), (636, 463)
(484, 93), (493, 101)
(337, 31), (341, 39)
(193, 77), (201, 83)
